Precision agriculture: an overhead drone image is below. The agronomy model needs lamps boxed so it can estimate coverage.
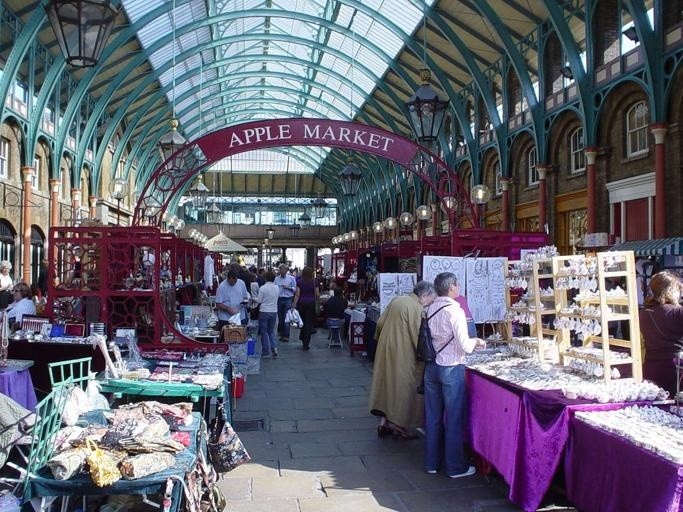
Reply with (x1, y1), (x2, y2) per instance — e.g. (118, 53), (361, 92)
(157, 0), (193, 172)
(406, 1), (451, 143)
(331, 231), (358, 245)
(297, 145), (312, 231)
(43, 1), (124, 68)
(372, 205), (432, 233)
(109, 178), (129, 225)
(145, 201), (209, 247)
(337, 13), (366, 196)
(440, 196), (459, 215)
(290, 158), (300, 237)
(313, 92), (329, 217)
(189, 50), (210, 208)
(206, 163), (223, 222)
(260, 225), (276, 251)
(470, 185), (490, 204)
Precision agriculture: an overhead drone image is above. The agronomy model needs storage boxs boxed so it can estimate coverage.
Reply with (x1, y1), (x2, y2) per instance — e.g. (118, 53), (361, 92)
(221, 323), (262, 399)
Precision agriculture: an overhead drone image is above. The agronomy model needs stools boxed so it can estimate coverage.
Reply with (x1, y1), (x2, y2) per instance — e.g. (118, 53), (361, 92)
(326, 317), (343, 350)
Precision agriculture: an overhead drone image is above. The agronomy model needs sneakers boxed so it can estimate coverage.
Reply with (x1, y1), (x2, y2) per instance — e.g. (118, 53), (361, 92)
(427, 469), (436, 474)
(261, 350), (278, 359)
(450, 466), (477, 478)
(278, 335), (290, 343)
(297, 336), (311, 349)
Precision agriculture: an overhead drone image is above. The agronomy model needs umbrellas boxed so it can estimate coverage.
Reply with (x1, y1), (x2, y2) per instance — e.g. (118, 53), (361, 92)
(199, 230), (249, 253)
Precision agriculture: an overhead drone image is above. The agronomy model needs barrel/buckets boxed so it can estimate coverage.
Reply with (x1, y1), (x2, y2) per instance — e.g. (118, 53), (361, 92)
(247, 337), (257, 355)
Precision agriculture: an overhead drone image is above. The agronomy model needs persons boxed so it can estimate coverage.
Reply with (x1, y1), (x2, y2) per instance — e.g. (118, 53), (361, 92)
(215, 261), (354, 359)
(453, 294), (477, 339)
(0, 260), (86, 330)
(423, 272), (476, 479)
(369, 281), (437, 440)
(639, 271), (683, 399)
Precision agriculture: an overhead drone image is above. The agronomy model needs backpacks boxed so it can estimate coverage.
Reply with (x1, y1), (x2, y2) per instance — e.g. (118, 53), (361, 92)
(414, 316), (438, 363)
(1, 287), (16, 310)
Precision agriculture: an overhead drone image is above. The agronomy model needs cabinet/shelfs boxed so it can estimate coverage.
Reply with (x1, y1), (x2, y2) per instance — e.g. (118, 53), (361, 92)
(550, 249), (643, 386)
(44, 223), (227, 355)
(331, 226), (551, 314)
(503, 256), (571, 364)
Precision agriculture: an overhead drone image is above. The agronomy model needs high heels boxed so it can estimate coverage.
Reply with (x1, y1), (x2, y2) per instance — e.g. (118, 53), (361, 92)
(376, 425), (392, 439)
(394, 428), (420, 442)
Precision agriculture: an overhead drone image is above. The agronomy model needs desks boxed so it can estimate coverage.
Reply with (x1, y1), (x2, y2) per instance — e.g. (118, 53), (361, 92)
(461, 343), (678, 511)
(0, 317), (233, 512)
(561, 399), (682, 511)
(343, 304), (378, 354)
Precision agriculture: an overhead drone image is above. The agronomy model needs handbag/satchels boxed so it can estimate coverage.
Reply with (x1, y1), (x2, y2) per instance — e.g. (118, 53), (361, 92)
(205, 420), (252, 476)
(249, 302), (260, 321)
